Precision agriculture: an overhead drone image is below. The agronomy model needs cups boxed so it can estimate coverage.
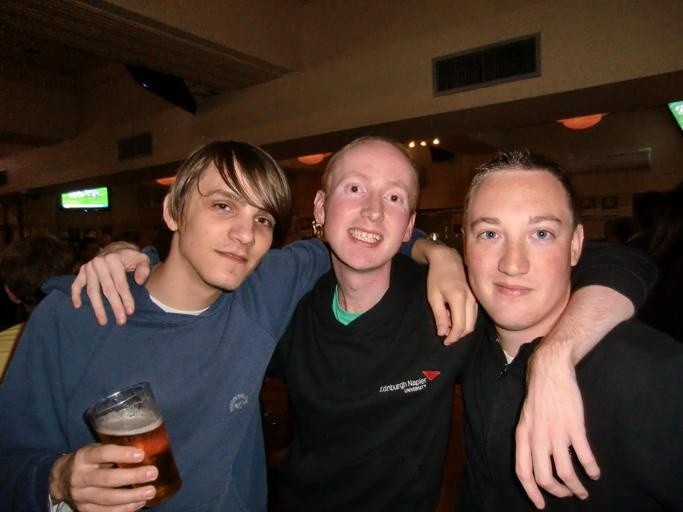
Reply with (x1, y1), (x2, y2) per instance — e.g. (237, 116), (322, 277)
(83, 380), (182, 502)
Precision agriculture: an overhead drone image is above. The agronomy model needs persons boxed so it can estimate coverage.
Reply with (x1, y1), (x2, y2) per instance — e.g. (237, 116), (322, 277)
(449, 145), (683, 511)
(0, 138), (479, 512)
(604, 184), (683, 338)
(2, 223), (172, 330)
(0, 231), (77, 383)
(69, 135), (663, 511)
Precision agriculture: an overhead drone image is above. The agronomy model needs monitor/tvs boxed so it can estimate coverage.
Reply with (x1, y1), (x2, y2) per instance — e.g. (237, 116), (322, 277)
(59, 186), (112, 213)
(667, 99), (682, 133)
